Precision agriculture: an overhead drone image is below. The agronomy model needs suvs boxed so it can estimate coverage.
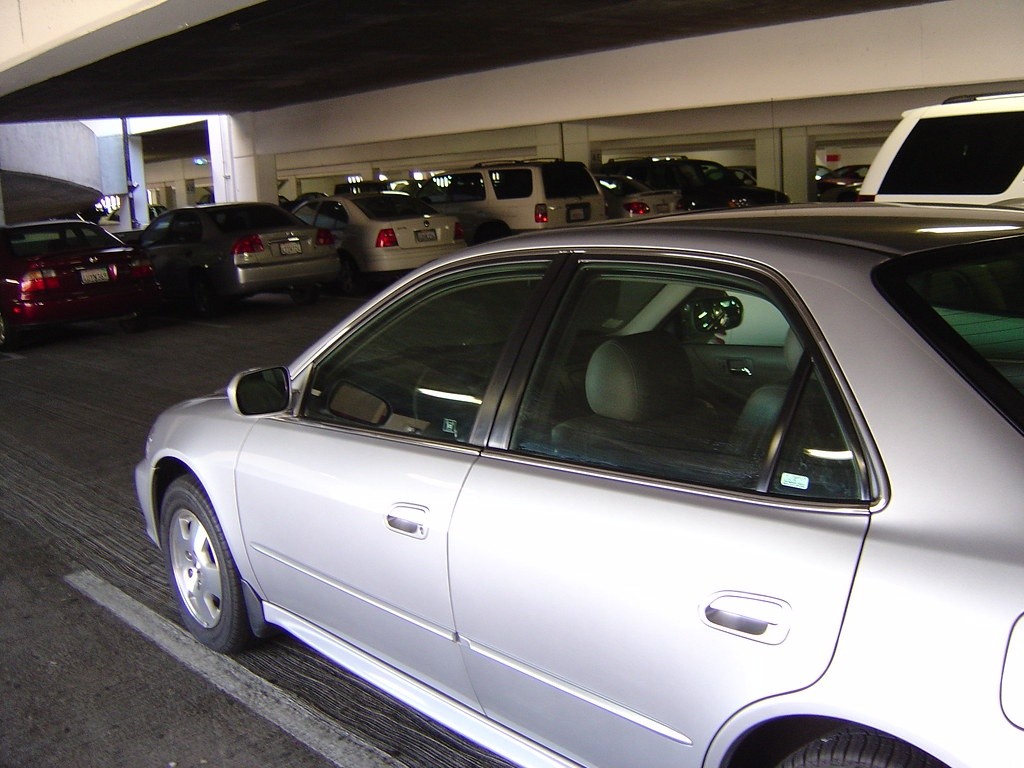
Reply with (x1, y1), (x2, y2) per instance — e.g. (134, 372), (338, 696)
(601, 156), (790, 214)
(413, 157), (610, 246)
(860, 91), (1024, 204)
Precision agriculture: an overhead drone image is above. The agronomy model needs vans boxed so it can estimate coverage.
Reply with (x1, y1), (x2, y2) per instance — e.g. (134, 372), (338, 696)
(334, 181), (393, 196)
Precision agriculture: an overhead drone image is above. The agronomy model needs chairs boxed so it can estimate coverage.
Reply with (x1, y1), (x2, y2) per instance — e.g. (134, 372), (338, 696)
(551, 330), (720, 484)
(722, 327), (829, 492)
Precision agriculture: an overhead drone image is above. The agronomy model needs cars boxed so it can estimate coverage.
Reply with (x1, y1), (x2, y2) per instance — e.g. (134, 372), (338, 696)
(97, 206), (169, 224)
(818, 164), (870, 197)
(131, 202), (340, 316)
(591, 174), (682, 220)
(0, 219), (153, 348)
(704, 165), (757, 186)
(816, 166), (832, 186)
(278, 191), (329, 211)
(133, 203), (1024, 768)
(290, 190), (470, 296)
